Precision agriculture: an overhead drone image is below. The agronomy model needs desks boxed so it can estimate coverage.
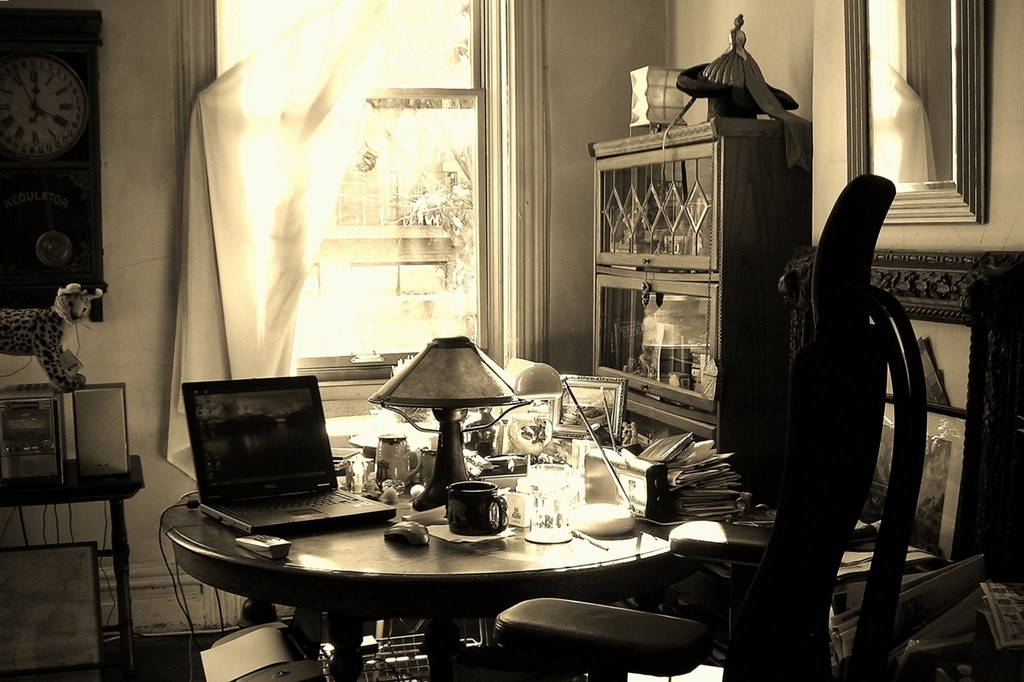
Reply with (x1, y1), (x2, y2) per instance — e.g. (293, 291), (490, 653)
(0, 456), (147, 679)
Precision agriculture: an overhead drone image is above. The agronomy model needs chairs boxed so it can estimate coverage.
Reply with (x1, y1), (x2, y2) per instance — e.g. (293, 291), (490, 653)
(494, 173), (923, 682)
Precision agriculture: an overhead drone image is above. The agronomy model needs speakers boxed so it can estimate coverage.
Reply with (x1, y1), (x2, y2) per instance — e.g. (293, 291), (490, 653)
(74, 381), (129, 478)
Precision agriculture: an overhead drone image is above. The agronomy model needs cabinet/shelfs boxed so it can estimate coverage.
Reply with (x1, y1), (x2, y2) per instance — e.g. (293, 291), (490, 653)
(584, 115), (813, 459)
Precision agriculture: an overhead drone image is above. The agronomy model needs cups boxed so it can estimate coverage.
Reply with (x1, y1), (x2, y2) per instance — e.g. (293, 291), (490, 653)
(447, 481), (509, 537)
(374, 434), (420, 485)
(419, 449), (438, 487)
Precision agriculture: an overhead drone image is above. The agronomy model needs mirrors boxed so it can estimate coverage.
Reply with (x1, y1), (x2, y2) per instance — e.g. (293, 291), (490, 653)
(844, 0), (989, 228)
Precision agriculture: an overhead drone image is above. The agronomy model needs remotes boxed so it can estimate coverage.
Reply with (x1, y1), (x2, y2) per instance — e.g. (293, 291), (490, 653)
(235, 535), (291, 558)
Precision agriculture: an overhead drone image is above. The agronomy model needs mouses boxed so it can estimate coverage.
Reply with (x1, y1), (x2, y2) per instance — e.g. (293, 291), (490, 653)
(384, 521), (428, 547)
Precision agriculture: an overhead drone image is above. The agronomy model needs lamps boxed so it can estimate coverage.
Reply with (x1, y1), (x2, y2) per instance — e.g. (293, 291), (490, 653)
(365, 336), (518, 513)
(628, 65), (687, 133)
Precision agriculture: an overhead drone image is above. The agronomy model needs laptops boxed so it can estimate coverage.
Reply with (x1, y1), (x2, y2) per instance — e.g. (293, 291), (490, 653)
(183, 376), (400, 533)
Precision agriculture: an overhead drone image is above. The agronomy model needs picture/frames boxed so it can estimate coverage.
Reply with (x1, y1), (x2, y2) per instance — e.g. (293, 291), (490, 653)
(781, 242), (1023, 573)
(548, 373), (627, 445)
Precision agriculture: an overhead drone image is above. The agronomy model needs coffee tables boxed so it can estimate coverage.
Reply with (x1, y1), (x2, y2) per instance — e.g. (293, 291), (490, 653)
(158, 438), (760, 682)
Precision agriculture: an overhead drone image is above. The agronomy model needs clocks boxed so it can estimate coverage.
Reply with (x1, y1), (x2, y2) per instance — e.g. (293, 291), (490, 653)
(0, 52), (91, 163)
